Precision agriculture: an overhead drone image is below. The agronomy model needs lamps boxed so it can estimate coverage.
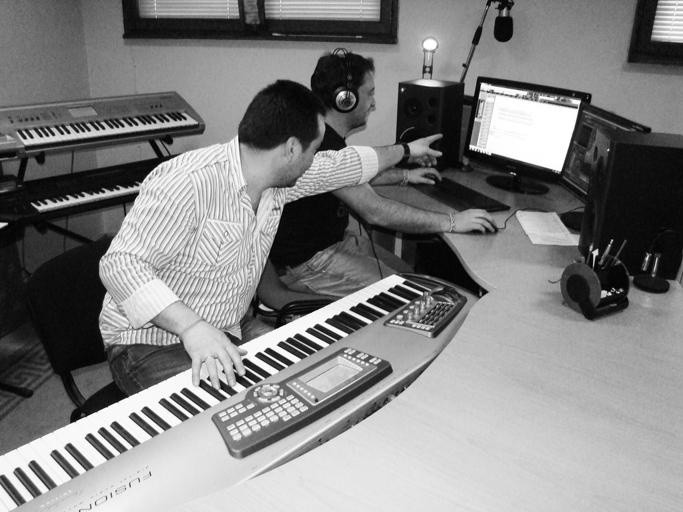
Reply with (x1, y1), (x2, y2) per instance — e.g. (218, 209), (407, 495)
(421, 37), (438, 79)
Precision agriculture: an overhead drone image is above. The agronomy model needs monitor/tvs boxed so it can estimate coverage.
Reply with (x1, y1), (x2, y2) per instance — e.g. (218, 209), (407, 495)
(558, 103), (651, 234)
(464, 76), (592, 195)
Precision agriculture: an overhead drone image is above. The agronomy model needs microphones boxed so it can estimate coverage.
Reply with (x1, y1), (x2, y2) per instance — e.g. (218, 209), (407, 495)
(494, 0), (515, 42)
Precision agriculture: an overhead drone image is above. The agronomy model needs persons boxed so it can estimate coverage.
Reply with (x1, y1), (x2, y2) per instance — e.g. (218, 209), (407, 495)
(268, 47), (498, 298)
(98, 80), (444, 405)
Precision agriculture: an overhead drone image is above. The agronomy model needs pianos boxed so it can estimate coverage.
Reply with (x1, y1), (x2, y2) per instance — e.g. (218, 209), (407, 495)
(1, 153), (181, 223)
(0, 272), (480, 512)
(0, 91), (206, 161)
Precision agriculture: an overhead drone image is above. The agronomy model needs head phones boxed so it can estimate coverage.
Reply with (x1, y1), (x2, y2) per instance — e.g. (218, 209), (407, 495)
(330, 48), (358, 113)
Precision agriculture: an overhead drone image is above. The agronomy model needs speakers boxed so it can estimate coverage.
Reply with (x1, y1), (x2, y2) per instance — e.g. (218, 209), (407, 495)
(578, 128), (683, 280)
(396, 78), (465, 171)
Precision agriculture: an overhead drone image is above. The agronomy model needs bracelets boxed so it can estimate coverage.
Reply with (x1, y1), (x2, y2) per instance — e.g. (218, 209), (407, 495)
(401, 167), (410, 187)
(395, 143), (411, 168)
(449, 211), (456, 234)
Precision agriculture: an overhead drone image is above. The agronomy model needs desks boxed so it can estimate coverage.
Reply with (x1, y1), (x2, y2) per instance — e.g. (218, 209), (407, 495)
(185, 163), (683, 512)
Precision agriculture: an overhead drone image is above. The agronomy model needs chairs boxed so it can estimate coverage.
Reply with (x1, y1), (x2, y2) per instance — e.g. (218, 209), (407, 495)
(27, 241), (128, 423)
(258, 260), (342, 329)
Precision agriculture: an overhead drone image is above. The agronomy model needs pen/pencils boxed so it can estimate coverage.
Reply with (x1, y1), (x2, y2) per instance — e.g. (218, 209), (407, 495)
(585, 238), (628, 270)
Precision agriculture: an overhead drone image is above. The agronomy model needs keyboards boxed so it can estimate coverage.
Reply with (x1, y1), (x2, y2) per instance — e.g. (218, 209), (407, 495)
(408, 177), (510, 212)
